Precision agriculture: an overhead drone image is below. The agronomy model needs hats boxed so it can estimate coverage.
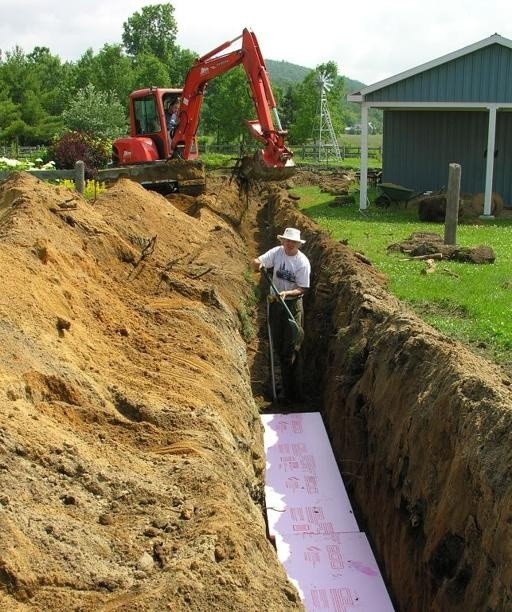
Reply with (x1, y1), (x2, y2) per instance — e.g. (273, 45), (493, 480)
(277, 228), (306, 245)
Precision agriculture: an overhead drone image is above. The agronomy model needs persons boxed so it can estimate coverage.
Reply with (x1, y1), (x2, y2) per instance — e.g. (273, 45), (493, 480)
(254, 227), (312, 369)
(154, 98), (181, 140)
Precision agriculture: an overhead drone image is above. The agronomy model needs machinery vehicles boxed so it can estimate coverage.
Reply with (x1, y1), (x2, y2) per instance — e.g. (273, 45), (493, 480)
(93, 26), (297, 195)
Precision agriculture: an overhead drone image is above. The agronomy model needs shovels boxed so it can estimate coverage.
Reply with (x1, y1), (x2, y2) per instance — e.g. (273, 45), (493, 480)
(260, 269), (305, 351)
